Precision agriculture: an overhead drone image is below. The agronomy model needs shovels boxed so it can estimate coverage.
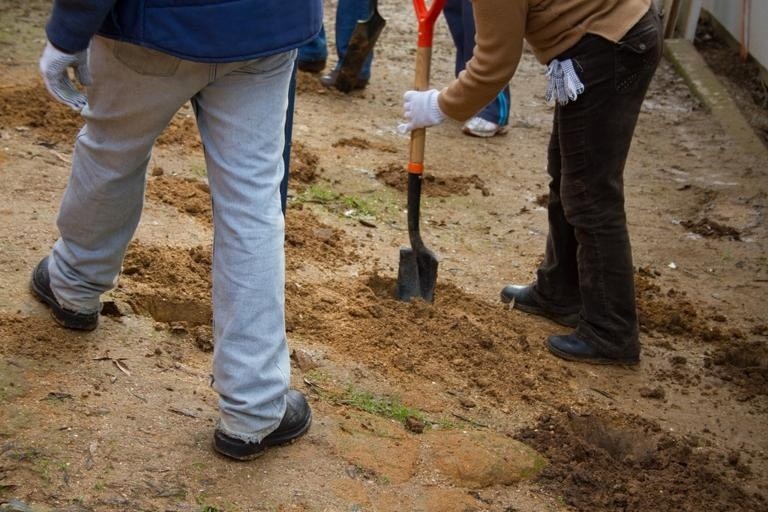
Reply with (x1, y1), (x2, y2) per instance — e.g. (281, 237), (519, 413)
(397, 0), (448, 305)
(335, 0), (388, 95)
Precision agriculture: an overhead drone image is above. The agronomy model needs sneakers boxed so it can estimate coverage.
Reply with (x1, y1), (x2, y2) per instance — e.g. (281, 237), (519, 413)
(461, 117), (511, 138)
(501, 283), (582, 329)
(320, 66), (369, 89)
(30, 254), (99, 331)
(214, 389), (311, 460)
(545, 328), (643, 366)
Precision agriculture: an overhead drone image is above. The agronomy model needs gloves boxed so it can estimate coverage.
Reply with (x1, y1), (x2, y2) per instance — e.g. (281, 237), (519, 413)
(396, 88), (447, 136)
(544, 59), (584, 107)
(38, 38), (94, 114)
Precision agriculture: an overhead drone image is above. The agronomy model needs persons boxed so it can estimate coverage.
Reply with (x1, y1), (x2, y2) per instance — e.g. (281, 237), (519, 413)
(29, 0), (323, 461)
(404, 0), (663, 366)
(320, 0), (511, 138)
